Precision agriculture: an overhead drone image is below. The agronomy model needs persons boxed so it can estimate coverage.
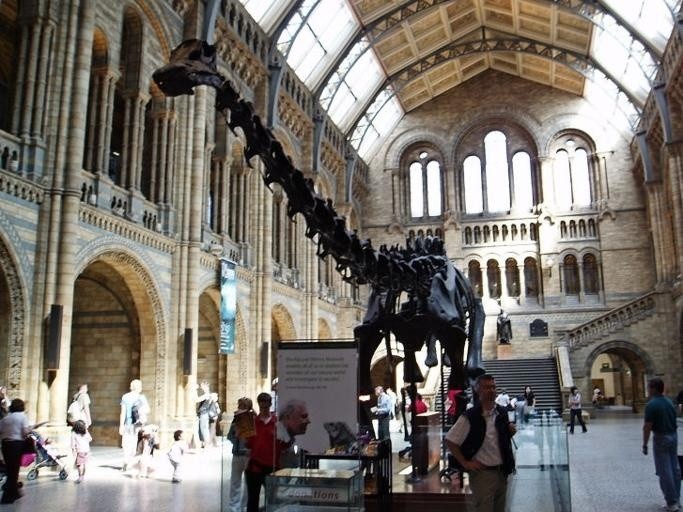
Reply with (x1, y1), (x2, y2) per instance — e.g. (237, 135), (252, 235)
(370, 367), (535, 440)
(569, 386), (587, 434)
(245, 393), (278, 512)
(227, 398), (256, 512)
(0, 379), (92, 504)
(643, 379), (681, 512)
(593, 386), (601, 395)
(497, 309), (512, 344)
(592, 391), (602, 409)
(275, 399), (311, 472)
(118, 379), (221, 482)
(446, 375), (516, 512)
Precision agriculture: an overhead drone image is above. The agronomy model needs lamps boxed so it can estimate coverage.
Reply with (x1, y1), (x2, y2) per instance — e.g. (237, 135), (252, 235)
(201, 241), (224, 255)
(547, 260), (555, 276)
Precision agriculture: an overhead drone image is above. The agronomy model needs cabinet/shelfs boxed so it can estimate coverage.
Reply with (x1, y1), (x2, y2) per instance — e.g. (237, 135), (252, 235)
(297, 437), (392, 512)
(264, 467), (366, 512)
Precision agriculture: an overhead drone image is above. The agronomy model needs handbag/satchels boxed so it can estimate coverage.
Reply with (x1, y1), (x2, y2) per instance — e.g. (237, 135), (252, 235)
(22, 437), (35, 455)
(234, 412), (257, 439)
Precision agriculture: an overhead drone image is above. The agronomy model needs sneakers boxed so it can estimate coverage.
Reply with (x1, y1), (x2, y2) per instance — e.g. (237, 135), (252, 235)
(662, 504), (678, 511)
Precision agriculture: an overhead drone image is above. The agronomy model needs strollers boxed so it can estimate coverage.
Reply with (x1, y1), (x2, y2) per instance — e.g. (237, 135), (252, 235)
(26, 431), (69, 480)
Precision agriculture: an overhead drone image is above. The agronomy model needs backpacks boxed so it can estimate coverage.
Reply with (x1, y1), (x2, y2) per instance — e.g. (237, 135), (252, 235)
(68, 393), (82, 422)
